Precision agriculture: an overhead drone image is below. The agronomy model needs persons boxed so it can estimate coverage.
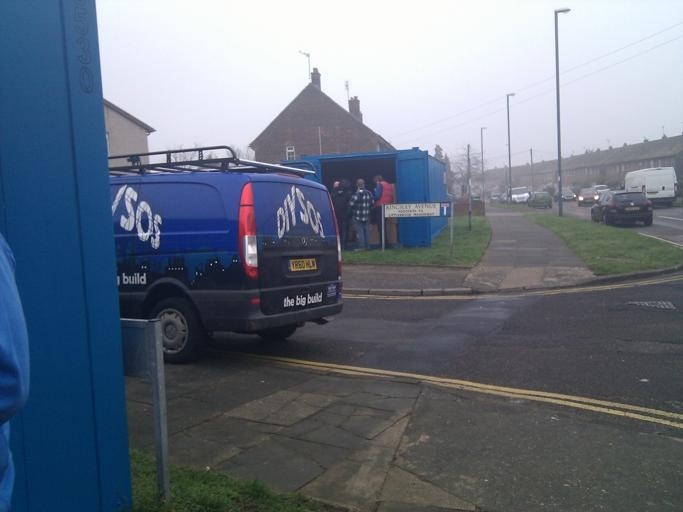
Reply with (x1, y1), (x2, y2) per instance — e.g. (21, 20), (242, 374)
(331, 175), (393, 251)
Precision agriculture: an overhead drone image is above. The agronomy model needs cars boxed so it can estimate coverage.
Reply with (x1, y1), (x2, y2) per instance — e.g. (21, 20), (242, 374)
(469, 184), (611, 208)
(590, 189), (654, 228)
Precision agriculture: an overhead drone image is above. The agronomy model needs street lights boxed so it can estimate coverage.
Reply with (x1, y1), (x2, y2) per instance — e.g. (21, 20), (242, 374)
(506, 93), (516, 204)
(480, 126), (487, 203)
(553, 7), (570, 218)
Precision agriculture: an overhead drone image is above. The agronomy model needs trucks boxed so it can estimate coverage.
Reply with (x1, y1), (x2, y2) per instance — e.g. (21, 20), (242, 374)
(619, 166), (678, 208)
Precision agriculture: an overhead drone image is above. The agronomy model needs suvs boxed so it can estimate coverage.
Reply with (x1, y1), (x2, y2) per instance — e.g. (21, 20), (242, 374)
(106, 145), (345, 361)
